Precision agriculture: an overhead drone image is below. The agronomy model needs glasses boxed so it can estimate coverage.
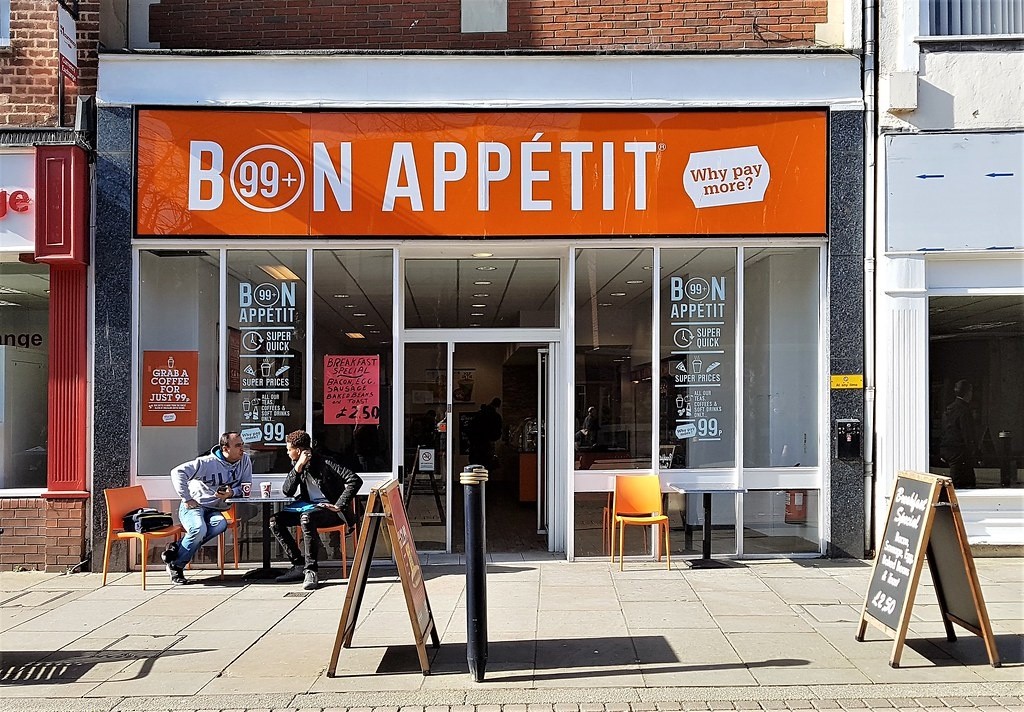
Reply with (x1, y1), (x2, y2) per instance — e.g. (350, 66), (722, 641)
(235, 442), (244, 447)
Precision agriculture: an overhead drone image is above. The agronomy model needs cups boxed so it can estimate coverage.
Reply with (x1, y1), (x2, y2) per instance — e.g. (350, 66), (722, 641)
(241, 483), (252, 498)
(259, 481), (272, 498)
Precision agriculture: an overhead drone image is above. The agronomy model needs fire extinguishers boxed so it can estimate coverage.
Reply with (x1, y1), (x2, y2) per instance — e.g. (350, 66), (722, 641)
(785, 462), (808, 524)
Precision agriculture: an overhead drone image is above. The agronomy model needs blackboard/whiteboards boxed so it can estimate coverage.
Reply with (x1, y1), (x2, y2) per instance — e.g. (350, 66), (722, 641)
(862, 470), (944, 638)
(659, 444), (676, 469)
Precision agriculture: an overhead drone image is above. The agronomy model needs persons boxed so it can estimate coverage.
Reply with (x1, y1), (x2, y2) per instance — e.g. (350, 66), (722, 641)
(574, 420), (596, 461)
(468, 397), (502, 494)
(269, 431), (363, 590)
(300, 395), (393, 472)
(158, 431), (253, 585)
(582, 406), (597, 450)
(417, 411), (437, 448)
(943, 379), (983, 489)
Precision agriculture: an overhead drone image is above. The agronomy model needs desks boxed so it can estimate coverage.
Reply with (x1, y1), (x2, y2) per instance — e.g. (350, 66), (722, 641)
(223, 494), (296, 583)
(666, 482), (748, 570)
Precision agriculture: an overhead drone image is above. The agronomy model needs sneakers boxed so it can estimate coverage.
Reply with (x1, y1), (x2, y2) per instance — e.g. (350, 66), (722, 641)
(275, 565), (305, 581)
(166, 561), (187, 585)
(303, 569), (319, 590)
(161, 541), (179, 564)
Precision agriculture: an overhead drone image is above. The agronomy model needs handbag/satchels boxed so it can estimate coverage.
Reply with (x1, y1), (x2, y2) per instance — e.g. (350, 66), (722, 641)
(122, 508), (173, 534)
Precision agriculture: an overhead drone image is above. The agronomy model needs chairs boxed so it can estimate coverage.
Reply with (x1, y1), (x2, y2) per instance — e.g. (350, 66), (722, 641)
(101, 485), (181, 593)
(602, 476), (650, 556)
(612, 475), (671, 573)
(296, 498), (357, 580)
(174, 503), (238, 581)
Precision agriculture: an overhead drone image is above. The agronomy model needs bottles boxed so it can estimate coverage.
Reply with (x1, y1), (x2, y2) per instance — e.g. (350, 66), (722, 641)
(432, 414), (446, 452)
